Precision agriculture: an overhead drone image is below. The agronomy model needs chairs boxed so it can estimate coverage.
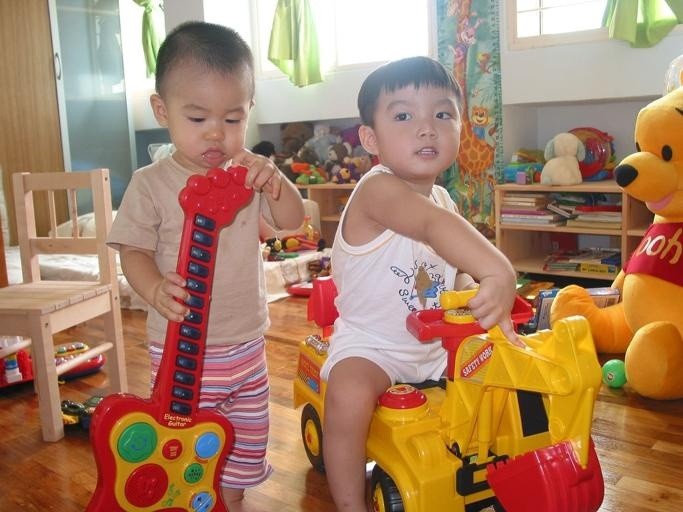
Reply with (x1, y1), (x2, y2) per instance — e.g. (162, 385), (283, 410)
(0, 167), (131, 447)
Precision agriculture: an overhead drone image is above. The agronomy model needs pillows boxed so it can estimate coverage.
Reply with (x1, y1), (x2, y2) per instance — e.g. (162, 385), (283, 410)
(46, 208), (123, 242)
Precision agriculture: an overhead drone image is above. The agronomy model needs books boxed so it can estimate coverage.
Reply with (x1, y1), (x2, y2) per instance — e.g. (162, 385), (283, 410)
(501, 191), (622, 275)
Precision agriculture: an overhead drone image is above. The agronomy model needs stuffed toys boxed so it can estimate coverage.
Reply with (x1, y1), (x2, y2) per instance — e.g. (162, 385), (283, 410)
(539, 132), (587, 186)
(548, 55), (682, 403)
(293, 142), (381, 185)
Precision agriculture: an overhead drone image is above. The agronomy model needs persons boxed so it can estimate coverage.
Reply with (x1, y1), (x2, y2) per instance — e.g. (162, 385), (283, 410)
(105, 22), (304, 512)
(318, 58), (528, 512)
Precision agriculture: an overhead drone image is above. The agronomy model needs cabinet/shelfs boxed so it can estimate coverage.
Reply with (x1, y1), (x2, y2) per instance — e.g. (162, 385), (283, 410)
(492, 177), (654, 286)
(294, 181), (361, 250)
